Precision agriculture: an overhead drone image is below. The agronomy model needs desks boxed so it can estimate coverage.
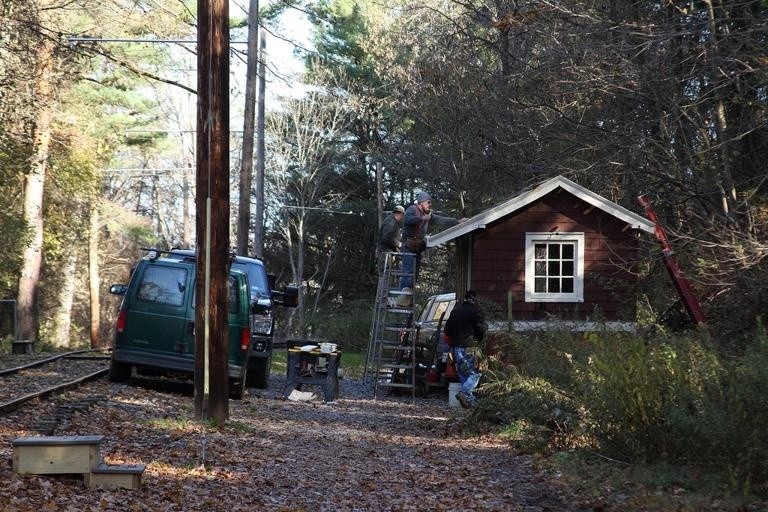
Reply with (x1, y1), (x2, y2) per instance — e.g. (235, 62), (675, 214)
(281, 344), (344, 403)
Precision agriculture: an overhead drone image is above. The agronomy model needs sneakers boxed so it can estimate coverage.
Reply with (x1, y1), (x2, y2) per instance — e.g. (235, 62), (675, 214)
(455, 391), (470, 409)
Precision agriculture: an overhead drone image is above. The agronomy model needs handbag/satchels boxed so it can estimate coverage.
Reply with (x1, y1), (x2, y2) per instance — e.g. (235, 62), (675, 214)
(404, 236), (426, 253)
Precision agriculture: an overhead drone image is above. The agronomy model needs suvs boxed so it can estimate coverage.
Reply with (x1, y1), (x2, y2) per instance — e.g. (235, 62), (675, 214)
(108, 247), (299, 401)
(392, 290), (459, 395)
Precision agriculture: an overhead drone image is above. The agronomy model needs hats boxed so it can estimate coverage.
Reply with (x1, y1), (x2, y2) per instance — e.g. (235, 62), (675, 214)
(393, 204), (406, 214)
(417, 190), (433, 202)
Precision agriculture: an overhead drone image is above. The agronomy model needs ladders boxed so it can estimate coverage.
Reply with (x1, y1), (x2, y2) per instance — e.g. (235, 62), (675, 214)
(364, 252), (417, 401)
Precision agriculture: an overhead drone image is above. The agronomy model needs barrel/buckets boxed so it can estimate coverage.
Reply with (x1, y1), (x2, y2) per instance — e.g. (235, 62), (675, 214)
(448, 383), (463, 407)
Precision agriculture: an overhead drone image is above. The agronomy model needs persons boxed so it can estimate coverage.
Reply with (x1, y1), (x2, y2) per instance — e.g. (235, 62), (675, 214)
(444, 290), (487, 410)
(397, 190), (468, 308)
(374, 205), (403, 307)
